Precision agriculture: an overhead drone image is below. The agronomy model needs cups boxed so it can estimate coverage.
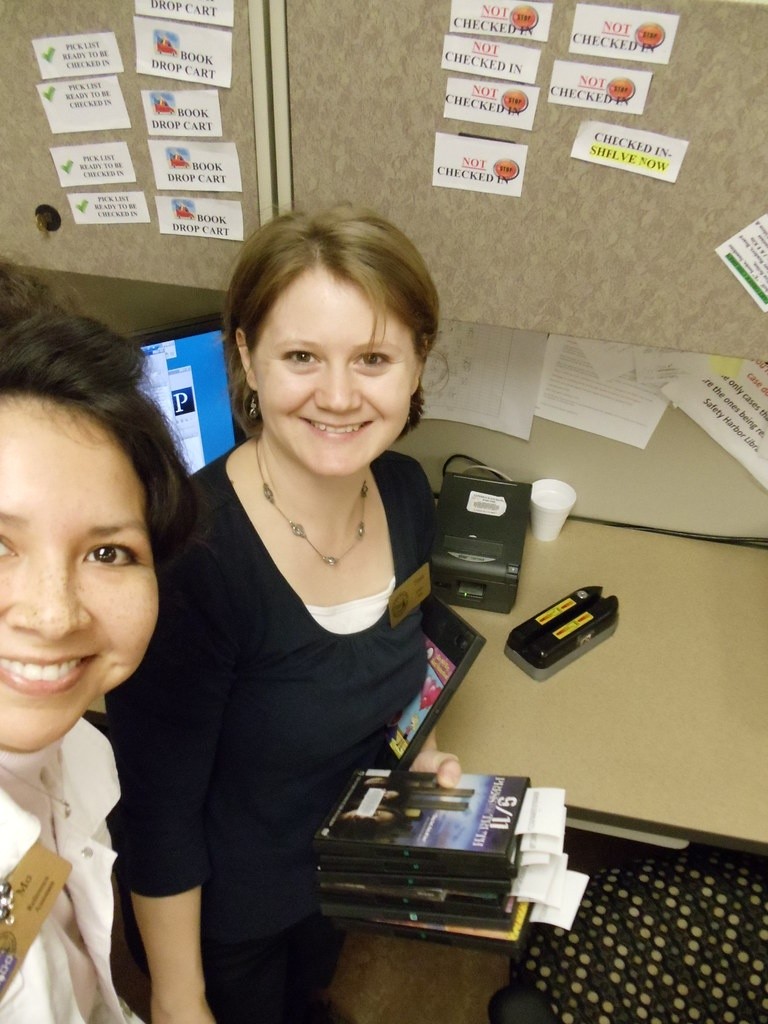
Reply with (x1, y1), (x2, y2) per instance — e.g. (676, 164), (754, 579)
(531, 479), (577, 542)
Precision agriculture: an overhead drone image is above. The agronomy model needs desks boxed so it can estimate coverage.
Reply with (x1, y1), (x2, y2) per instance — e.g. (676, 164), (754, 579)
(411, 495), (768, 854)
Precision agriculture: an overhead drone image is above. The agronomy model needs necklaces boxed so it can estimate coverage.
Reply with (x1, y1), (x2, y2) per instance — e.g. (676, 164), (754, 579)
(0, 763), (70, 819)
(255, 438), (369, 565)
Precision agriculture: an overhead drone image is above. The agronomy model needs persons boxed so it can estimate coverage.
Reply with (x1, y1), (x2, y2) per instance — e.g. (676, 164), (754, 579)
(102, 202), (440, 1024)
(0, 297), (192, 1024)
(336, 777), (408, 837)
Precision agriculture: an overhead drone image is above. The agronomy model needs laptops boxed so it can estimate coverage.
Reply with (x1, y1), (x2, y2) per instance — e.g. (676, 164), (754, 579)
(124, 310), (250, 476)
